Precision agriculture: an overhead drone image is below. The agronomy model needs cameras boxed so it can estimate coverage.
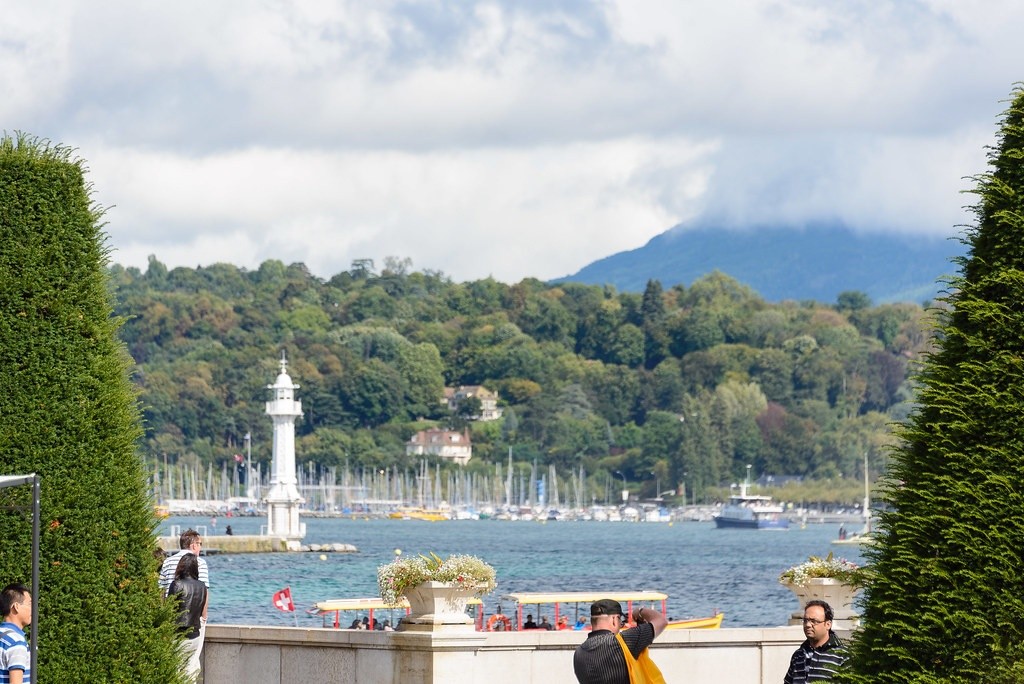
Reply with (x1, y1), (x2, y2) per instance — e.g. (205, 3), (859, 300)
(637, 618), (646, 626)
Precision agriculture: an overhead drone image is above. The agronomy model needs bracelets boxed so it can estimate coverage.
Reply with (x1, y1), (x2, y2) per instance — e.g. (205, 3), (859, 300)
(639, 606), (646, 618)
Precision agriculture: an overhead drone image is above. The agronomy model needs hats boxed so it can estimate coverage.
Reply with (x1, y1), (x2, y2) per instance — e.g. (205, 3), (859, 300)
(591, 599), (628, 619)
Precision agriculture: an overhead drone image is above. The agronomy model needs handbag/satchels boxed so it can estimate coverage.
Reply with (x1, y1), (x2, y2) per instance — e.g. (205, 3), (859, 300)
(616, 633), (666, 684)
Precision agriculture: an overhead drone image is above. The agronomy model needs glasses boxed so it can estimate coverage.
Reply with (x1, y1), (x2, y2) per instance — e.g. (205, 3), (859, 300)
(801, 618), (825, 625)
(617, 616), (625, 628)
(196, 541), (203, 545)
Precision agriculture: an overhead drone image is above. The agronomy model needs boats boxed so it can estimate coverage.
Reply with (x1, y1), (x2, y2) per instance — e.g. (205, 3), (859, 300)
(714, 478), (792, 529)
(305, 590), (725, 630)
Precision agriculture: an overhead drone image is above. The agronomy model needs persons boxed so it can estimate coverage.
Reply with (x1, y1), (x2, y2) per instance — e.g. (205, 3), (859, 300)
(522, 612), (642, 631)
(574, 599), (668, 683)
(158, 528), (210, 674)
(166, 553), (207, 684)
(346, 617), (403, 631)
(781, 601), (854, 684)
(0, 583), (33, 684)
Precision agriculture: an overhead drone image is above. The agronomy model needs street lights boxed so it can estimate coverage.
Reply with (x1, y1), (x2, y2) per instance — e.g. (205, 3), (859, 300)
(0, 473), (39, 684)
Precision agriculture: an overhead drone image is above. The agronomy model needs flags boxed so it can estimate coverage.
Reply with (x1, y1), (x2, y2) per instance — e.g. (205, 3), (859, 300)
(273, 587), (294, 613)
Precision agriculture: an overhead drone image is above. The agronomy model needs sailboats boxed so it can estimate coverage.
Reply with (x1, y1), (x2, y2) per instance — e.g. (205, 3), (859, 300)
(829, 450), (883, 545)
(142, 433), (824, 524)
